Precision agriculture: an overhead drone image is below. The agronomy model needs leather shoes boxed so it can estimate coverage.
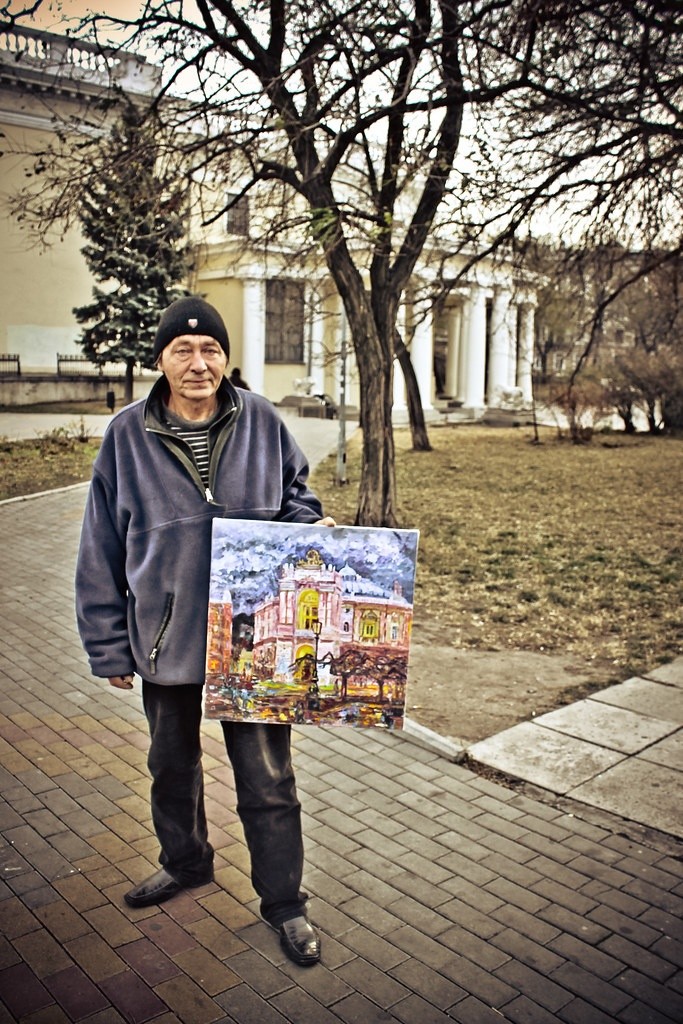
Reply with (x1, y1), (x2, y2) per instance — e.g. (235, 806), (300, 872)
(125, 865), (215, 909)
(279, 915), (321, 965)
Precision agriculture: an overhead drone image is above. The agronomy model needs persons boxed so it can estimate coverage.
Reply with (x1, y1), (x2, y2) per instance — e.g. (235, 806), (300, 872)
(227, 367), (250, 391)
(75, 297), (338, 966)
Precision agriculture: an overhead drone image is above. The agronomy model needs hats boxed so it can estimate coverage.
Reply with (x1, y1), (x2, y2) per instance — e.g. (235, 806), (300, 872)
(153, 297), (230, 363)
